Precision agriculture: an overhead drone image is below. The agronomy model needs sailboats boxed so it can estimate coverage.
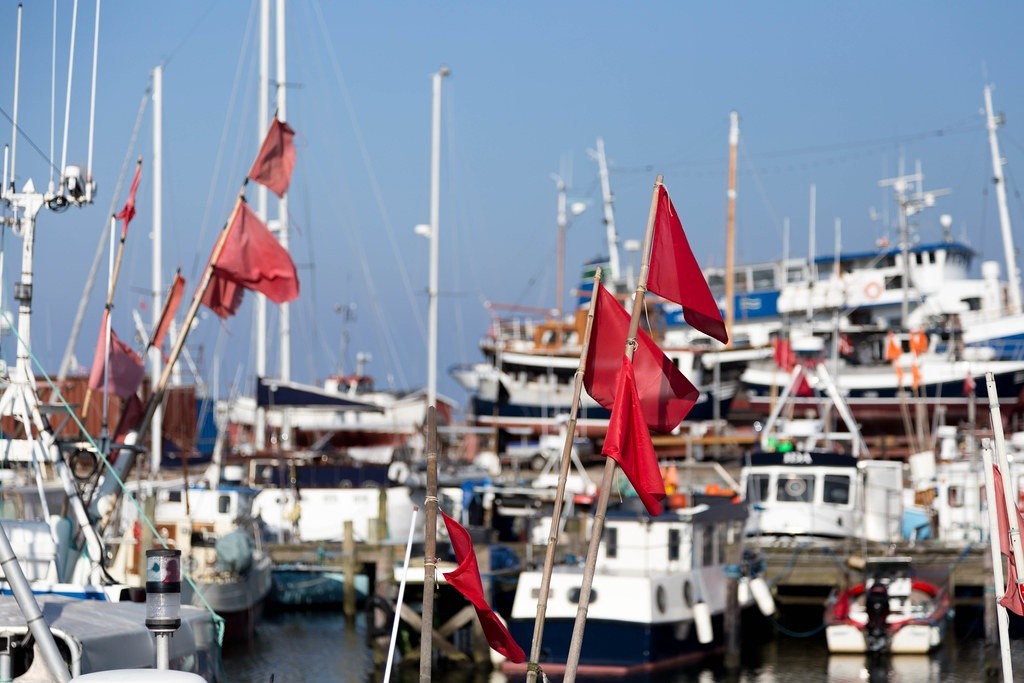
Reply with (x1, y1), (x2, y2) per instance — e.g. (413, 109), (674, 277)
(0, 0), (1024, 682)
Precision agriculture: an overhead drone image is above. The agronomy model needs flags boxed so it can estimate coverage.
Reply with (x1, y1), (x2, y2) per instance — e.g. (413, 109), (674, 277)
(249, 119), (297, 198)
(603, 353), (666, 515)
(195, 203), (300, 317)
(440, 510), (526, 664)
(583, 281), (701, 434)
(647, 185), (729, 345)
(993, 464), (1024, 617)
(780, 340), (813, 395)
(883, 328), (927, 392)
(89, 311), (145, 401)
(115, 169), (141, 237)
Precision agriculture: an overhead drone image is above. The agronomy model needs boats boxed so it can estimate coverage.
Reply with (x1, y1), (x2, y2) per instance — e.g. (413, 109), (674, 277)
(825, 574), (952, 656)
